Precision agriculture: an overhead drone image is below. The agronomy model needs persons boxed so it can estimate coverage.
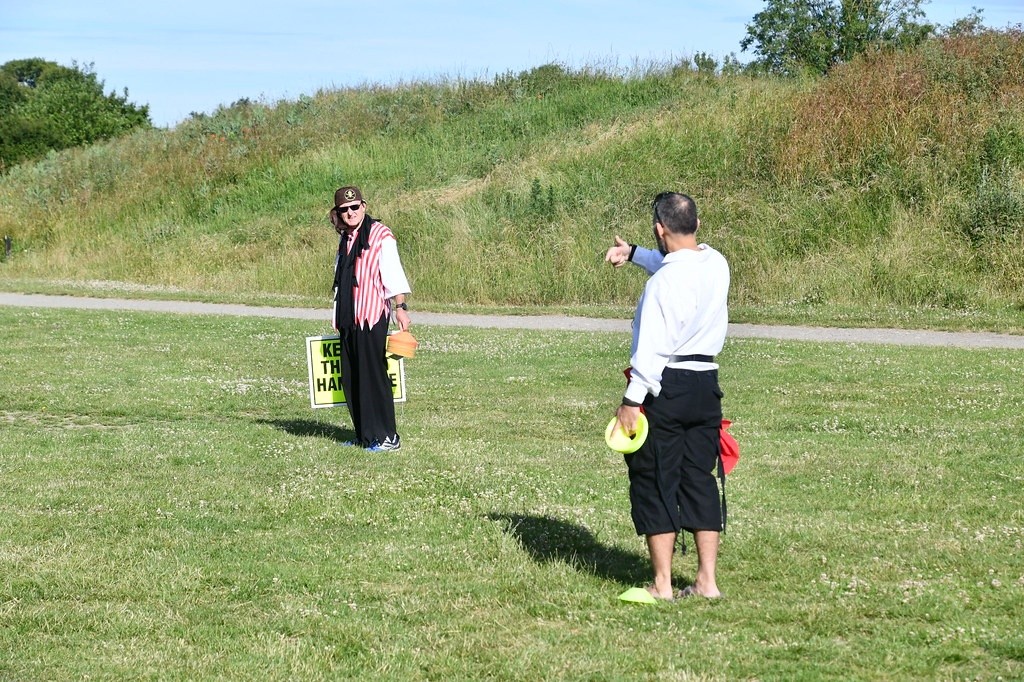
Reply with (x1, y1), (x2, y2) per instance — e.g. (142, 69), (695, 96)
(605, 193), (731, 605)
(328, 185), (412, 454)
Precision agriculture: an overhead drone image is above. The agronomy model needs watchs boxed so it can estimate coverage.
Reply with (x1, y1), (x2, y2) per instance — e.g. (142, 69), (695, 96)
(396, 302), (407, 310)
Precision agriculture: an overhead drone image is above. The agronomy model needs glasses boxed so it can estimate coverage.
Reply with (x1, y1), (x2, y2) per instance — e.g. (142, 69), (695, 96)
(337, 202), (363, 213)
(654, 191), (674, 228)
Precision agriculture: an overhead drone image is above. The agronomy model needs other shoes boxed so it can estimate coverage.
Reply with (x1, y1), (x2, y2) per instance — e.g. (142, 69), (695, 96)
(673, 583), (723, 601)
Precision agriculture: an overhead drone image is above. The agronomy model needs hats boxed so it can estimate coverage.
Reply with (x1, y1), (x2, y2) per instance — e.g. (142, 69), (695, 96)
(334, 186), (362, 206)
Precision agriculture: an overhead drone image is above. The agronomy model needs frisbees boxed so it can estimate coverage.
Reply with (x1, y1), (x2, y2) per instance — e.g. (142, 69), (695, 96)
(604, 412), (648, 454)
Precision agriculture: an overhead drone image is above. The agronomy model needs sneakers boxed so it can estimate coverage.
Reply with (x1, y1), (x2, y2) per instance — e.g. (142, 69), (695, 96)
(363, 432), (402, 452)
(341, 436), (366, 447)
